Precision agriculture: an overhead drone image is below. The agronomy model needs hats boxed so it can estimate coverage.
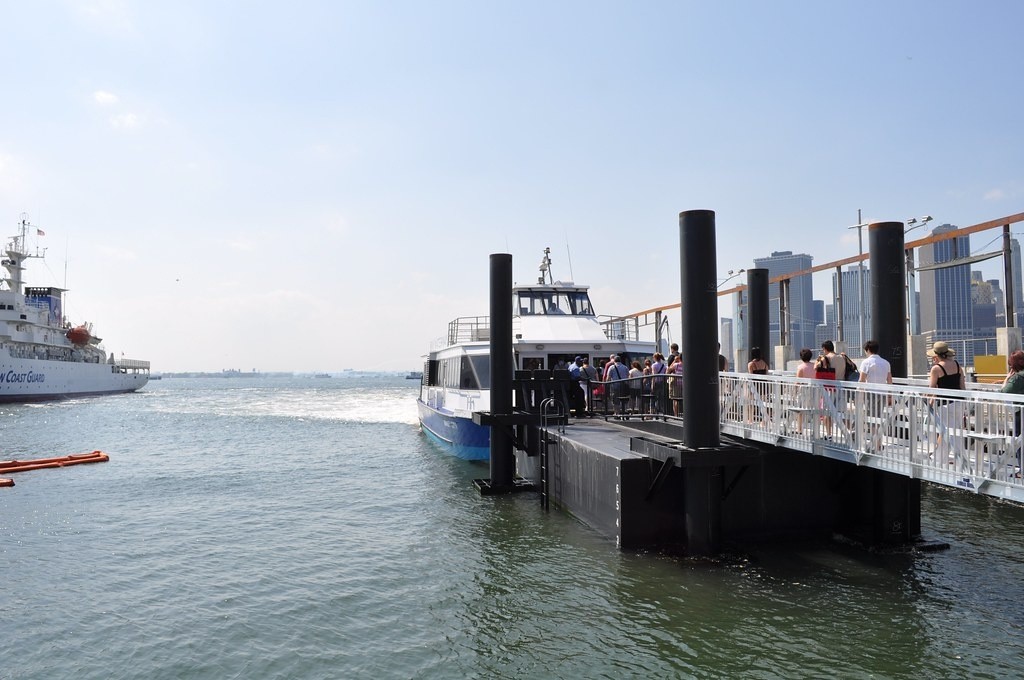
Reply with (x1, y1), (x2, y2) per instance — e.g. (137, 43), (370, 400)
(926, 341), (955, 358)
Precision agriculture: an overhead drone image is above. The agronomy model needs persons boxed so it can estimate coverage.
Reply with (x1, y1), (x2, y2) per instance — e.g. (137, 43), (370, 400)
(548, 302), (565, 316)
(857, 339), (894, 451)
(551, 343), (684, 421)
(926, 341), (967, 465)
(792, 348), (819, 436)
(746, 346), (771, 429)
(923, 349), (949, 459)
(719, 340), (731, 371)
(1001, 349), (1023, 477)
(814, 341), (858, 443)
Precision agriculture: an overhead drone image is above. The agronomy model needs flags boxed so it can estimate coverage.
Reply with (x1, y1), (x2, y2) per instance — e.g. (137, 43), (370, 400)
(37, 229), (45, 236)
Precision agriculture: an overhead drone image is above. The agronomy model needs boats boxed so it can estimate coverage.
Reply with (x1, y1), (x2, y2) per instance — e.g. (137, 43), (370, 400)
(0, 214), (150, 405)
(406, 372), (422, 379)
(416, 248), (672, 462)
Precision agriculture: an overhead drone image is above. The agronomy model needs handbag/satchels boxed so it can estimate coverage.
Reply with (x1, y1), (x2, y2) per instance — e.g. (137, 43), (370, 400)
(816, 356), (836, 391)
